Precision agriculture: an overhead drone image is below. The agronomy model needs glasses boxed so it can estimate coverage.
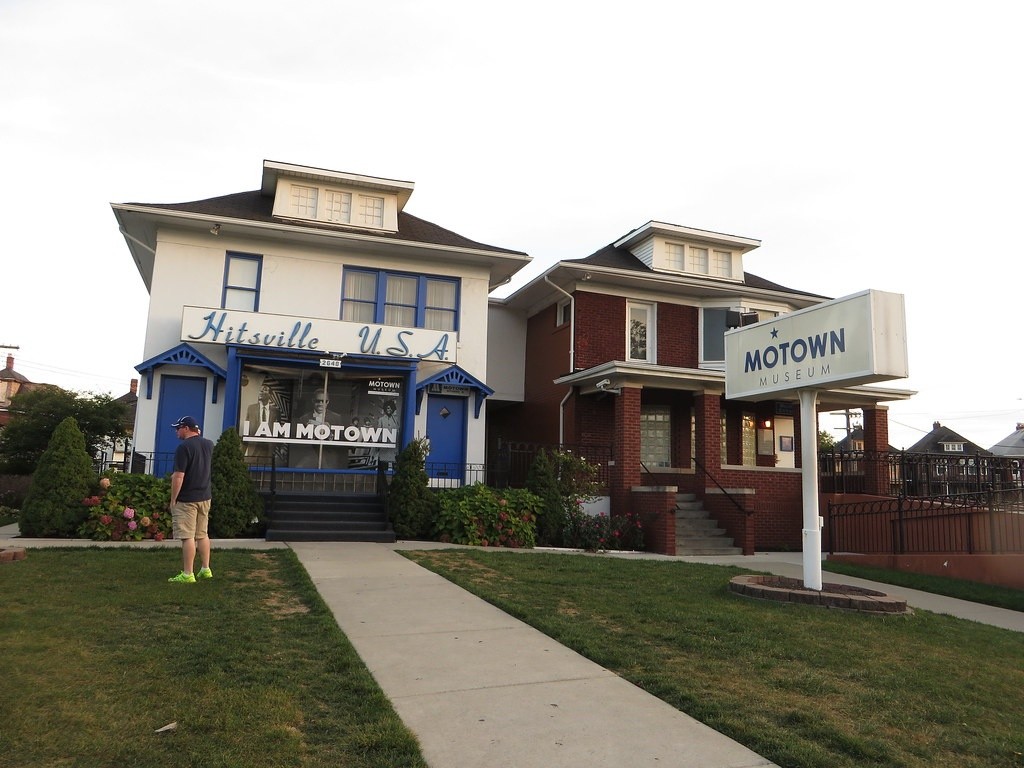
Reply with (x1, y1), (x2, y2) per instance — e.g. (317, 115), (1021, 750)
(175, 425), (189, 430)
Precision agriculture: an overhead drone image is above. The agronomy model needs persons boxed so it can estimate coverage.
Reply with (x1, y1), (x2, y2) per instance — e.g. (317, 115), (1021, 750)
(168, 416), (214, 582)
(241, 385), (286, 461)
(364, 418), (371, 427)
(299, 389), (341, 440)
(348, 417), (360, 433)
(376, 401), (400, 488)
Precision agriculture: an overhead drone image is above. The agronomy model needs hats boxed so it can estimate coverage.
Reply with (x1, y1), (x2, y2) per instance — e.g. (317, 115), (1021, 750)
(170, 415), (195, 427)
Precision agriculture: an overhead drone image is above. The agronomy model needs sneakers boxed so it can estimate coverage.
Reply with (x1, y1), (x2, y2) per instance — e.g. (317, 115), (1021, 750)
(168, 570), (197, 582)
(196, 568), (213, 578)
